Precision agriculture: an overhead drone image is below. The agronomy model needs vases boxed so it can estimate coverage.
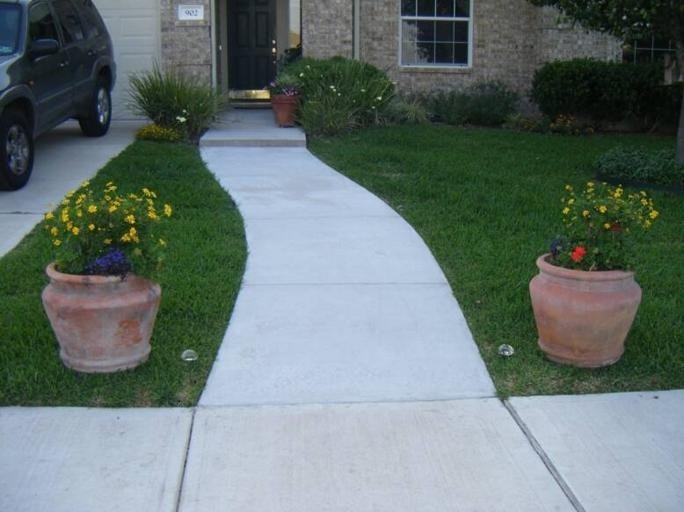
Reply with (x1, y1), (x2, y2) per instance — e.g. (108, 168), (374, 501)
(272, 94), (303, 125)
(41, 259), (162, 374)
(529, 251), (643, 370)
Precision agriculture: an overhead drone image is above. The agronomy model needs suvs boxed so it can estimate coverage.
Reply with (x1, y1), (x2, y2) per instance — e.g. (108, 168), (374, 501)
(0, 1), (116, 191)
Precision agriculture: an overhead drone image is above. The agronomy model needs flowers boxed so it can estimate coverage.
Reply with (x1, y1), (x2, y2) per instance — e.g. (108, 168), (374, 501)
(43, 177), (176, 281)
(545, 181), (660, 270)
(267, 74), (305, 98)
